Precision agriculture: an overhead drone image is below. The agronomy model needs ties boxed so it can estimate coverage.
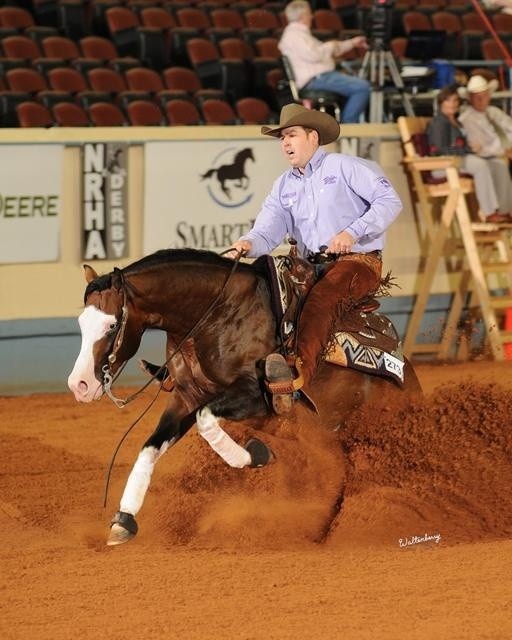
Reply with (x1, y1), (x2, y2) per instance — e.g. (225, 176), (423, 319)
(484, 110), (511, 153)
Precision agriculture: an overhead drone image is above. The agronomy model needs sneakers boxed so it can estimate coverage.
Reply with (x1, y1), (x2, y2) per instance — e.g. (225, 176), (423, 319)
(484, 210), (512, 224)
(263, 351), (296, 415)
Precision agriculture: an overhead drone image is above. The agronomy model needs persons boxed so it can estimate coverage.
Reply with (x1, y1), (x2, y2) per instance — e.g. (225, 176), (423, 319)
(457, 75), (512, 164)
(425, 87), (512, 224)
(138, 103), (404, 416)
(275, 0), (372, 123)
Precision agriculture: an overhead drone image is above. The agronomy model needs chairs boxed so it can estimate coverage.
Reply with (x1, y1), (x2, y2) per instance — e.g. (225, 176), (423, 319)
(1, 1), (512, 128)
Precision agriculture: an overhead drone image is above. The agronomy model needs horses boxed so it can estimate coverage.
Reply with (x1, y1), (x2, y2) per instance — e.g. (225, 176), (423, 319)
(65, 247), (427, 550)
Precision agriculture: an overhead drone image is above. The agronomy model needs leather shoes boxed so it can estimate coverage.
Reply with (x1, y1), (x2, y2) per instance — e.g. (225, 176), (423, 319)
(138, 357), (170, 382)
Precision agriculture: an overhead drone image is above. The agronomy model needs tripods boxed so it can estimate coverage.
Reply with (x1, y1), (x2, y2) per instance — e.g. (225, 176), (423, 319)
(358, 46), (417, 122)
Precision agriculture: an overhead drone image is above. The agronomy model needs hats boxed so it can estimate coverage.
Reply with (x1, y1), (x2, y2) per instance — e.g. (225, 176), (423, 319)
(456, 73), (500, 100)
(260, 102), (341, 146)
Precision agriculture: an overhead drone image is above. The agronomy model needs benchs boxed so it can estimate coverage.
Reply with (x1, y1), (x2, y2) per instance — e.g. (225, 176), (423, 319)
(395, 115), (512, 223)
